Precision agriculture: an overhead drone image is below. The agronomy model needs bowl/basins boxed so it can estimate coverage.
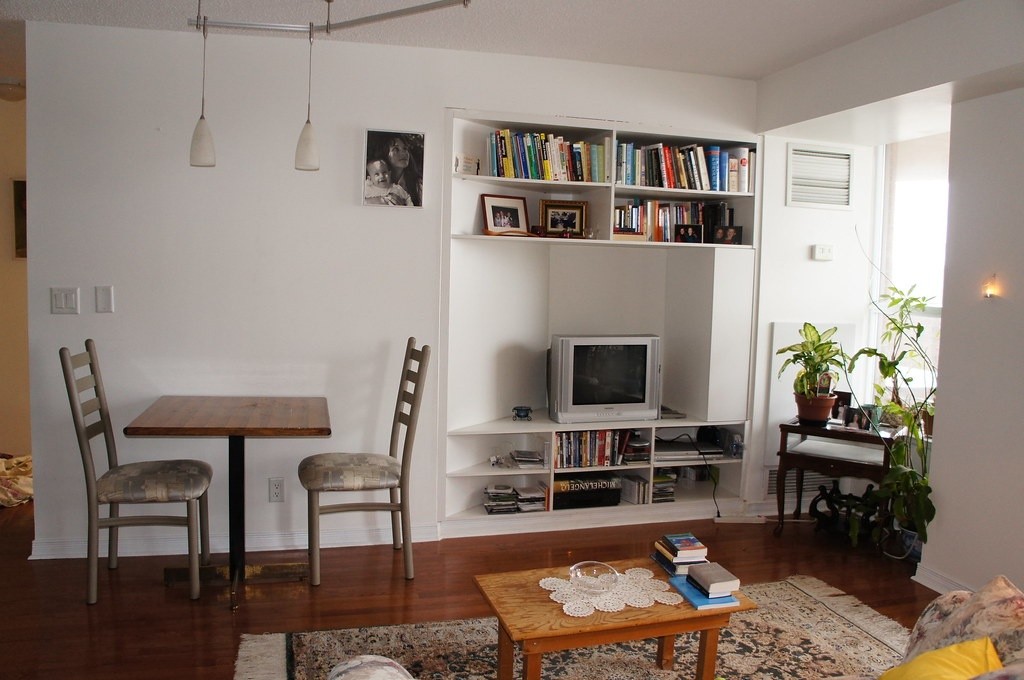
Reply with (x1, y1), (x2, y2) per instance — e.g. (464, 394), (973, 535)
(569, 560), (619, 596)
(582, 230), (599, 240)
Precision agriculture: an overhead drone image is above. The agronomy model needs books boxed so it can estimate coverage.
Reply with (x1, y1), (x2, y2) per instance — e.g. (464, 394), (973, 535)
(616, 140), (756, 193)
(613, 195), (734, 241)
(622, 475), (649, 504)
(483, 480), (550, 515)
(486, 129), (610, 183)
(555, 430), (651, 469)
(509, 436), (550, 469)
(650, 532), (740, 611)
(652, 476), (675, 503)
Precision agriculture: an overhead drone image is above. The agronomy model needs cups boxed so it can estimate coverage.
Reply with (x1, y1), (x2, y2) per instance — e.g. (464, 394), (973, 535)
(531, 225), (547, 236)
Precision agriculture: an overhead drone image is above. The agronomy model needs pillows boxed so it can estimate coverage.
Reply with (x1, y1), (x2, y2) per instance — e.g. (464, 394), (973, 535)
(878, 574), (1024, 680)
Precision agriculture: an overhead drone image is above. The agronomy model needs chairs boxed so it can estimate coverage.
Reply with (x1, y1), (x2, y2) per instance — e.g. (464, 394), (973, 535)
(58, 339), (213, 603)
(299, 337), (432, 586)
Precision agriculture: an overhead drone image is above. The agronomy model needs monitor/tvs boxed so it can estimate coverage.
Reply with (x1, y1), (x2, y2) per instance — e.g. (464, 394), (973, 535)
(549, 334), (663, 423)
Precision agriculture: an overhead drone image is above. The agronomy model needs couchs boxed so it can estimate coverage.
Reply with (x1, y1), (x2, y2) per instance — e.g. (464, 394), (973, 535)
(329, 592), (1024, 680)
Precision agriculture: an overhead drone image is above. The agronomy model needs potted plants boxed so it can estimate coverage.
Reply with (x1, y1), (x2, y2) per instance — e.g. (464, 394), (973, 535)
(776, 323), (852, 427)
(847, 284), (950, 564)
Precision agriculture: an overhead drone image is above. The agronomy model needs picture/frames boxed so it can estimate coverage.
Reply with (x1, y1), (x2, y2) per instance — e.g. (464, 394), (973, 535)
(540, 199), (589, 238)
(481, 193), (531, 236)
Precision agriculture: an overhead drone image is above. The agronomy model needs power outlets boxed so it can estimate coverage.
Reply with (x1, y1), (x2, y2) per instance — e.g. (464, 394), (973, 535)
(268, 478), (285, 502)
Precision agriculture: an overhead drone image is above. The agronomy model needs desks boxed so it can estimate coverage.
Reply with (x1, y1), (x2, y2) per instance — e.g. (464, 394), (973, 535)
(128, 397), (333, 610)
(472, 555), (758, 680)
(773, 413), (905, 538)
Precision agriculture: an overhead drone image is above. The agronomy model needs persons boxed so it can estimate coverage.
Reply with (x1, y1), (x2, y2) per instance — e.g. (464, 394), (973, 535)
(686, 227), (698, 243)
(365, 159), (414, 206)
(382, 135), (423, 206)
(714, 227), (725, 244)
(676, 226), (687, 242)
(495, 210), (513, 228)
(723, 227), (738, 244)
(848, 410), (870, 429)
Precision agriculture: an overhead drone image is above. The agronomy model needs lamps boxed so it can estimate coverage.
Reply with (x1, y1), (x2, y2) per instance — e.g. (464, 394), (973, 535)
(189, 18), (216, 165)
(294, 23), (321, 170)
(982, 274), (1004, 299)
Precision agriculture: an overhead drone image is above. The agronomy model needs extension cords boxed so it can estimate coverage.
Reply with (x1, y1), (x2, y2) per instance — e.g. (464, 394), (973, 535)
(714, 516), (767, 523)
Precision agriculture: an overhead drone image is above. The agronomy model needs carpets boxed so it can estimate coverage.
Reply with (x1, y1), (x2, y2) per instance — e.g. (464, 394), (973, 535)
(233, 577), (912, 680)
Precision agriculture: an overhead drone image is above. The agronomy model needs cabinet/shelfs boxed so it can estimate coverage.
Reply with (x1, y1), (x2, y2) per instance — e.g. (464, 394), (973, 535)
(439, 106), (764, 540)
